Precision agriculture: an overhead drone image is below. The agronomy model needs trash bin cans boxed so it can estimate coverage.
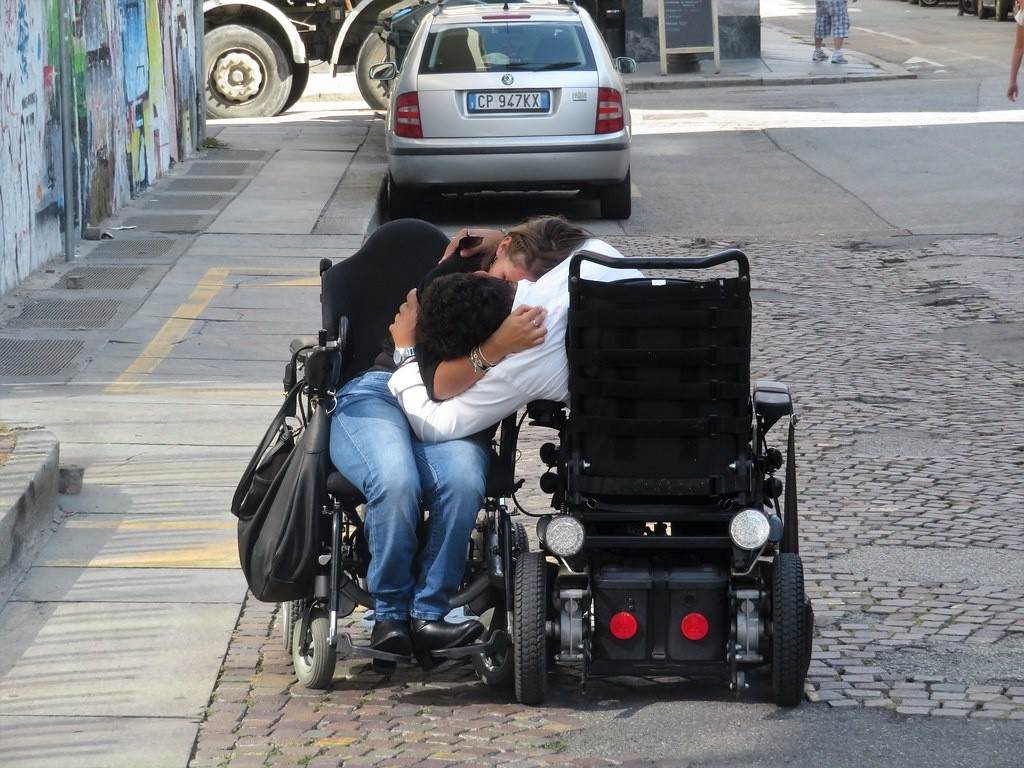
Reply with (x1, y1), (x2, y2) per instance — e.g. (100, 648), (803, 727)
(600, 9), (624, 60)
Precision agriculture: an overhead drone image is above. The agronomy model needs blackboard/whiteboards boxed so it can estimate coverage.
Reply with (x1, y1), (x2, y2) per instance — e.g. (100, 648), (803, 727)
(658, 0), (720, 55)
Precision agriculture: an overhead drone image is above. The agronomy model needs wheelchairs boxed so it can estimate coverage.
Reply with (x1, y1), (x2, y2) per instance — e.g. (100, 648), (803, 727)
(284, 257), (530, 692)
(499, 250), (816, 709)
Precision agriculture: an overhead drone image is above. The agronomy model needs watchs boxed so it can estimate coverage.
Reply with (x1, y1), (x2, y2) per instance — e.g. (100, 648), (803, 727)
(393, 347), (416, 366)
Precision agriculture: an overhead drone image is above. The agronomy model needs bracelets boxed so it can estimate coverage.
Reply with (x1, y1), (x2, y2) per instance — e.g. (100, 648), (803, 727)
(469, 346), (492, 372)
(501, 228), (509, 237)
(478, 342), (496, 366)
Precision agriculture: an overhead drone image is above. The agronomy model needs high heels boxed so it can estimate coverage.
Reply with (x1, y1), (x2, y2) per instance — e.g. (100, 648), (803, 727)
(410, 619), (485, 670)
(370, 620), (411, 672)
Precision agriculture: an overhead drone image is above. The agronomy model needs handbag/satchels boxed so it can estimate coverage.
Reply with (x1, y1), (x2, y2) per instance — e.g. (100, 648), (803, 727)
(231, 377), (333, 603)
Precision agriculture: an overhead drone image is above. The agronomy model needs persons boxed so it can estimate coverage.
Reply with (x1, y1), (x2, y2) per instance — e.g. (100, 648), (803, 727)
(415, 221), (645, 411)
(812, 0), (850, 64)
(320, 217), (589, 675)
(1007, 0), (1024, 102)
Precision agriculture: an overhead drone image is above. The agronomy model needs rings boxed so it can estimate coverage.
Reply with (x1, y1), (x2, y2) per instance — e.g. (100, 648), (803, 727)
(532, 319), (538, 326)
(467, 228), (471, 235)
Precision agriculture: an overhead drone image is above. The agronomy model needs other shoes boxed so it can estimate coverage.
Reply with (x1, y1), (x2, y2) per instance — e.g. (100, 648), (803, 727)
(831, 53), (848, 64)
(813, 49), (829, 61)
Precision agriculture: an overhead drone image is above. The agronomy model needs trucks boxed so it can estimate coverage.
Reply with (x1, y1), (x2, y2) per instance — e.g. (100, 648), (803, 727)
(202, 0), (530, 121)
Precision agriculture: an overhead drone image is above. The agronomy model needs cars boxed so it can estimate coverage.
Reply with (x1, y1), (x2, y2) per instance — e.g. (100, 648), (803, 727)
(385, 1), (636, 226)
